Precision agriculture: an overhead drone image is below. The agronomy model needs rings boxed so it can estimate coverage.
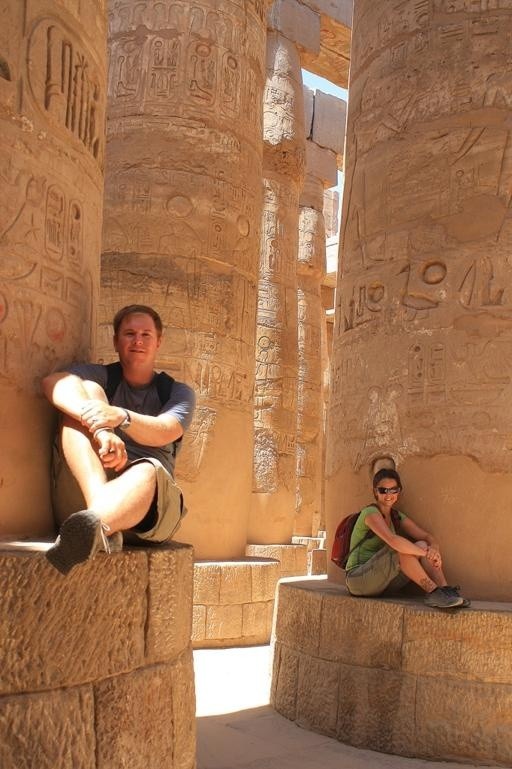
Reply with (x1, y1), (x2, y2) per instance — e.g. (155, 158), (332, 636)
(108, 446), (115, 454)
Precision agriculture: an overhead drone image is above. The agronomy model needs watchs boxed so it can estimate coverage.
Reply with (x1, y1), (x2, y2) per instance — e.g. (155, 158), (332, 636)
(116, 407), (131, 430)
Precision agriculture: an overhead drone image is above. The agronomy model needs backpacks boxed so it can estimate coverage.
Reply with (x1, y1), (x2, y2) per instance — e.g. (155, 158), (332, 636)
(330, 503), (402, 569)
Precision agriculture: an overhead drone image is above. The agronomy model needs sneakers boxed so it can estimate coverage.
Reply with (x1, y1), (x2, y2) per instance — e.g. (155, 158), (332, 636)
(101, 531), (124, 553)
(440, 586), (471, 607)
(44, 510), (100, 576)
(423, 586), (463, 609)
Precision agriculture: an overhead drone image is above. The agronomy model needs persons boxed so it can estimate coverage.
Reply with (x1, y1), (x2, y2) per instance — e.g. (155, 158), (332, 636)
(331, 468), (471, 610)
(31, 303), (197, 574)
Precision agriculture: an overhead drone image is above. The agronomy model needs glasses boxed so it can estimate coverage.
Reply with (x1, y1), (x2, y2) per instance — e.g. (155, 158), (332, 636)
(374, 485), (401, 495)
